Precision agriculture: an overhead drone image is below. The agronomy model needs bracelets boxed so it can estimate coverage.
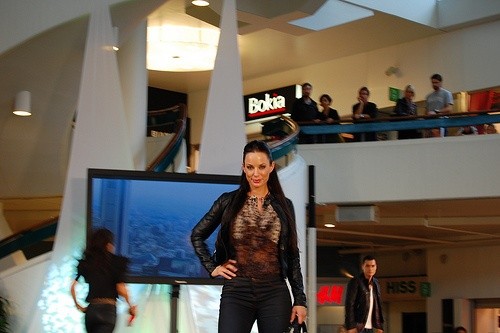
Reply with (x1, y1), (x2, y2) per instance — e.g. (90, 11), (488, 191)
(433, 109), (440, 114)
(360, 114), (364, 118)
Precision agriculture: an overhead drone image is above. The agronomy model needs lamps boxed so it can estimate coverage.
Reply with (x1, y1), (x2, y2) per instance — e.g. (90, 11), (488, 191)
(146, 23), (220, 71)
(12, 90), (31, 116)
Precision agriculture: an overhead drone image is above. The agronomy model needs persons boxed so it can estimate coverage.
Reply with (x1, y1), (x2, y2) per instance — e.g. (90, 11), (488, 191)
(71, 227), (135, 333)
(191, 140), (307, 333)
(425, 74), (454, 138)
(352, 87), (377, 142)
(453, 114), (497, 136)
(345, 256), (384, 333)
(291, 83), (320, 144)
(314, 94), (340, 143)
(394, 84), (422, 140)
